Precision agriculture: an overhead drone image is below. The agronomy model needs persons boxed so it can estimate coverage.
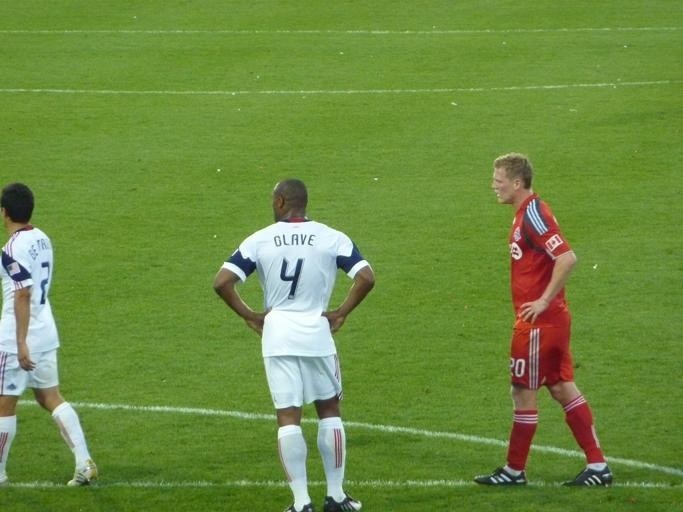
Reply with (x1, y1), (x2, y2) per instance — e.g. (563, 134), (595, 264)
(0, 183), (99, 486)
(213, 177), (377, 512)
(473, 153), (614, 489)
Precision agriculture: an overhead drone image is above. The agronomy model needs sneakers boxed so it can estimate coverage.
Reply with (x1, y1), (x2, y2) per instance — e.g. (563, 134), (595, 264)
(322, 491), (362, 511)
(66, 459), (97, 488)
(283, 503), (315, 511)
(563, 464), (613, 486)
(473, 466), (528, 485)
